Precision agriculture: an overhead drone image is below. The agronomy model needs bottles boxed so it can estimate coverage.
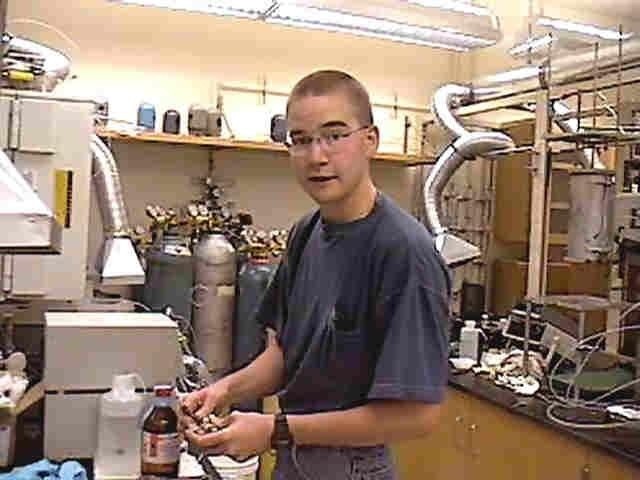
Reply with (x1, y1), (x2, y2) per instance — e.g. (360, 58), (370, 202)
(93, 374), (144, 480)
(141, 385), (181, 478)
(460, 320), (478, 366)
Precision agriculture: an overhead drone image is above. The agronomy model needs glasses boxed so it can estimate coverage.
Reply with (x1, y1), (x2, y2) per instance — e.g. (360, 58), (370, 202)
(284, 128), (368, 153)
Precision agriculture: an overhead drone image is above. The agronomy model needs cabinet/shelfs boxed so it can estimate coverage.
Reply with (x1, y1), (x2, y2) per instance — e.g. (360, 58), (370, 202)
(493, 258), (610, 338)
(386, 370), (640, 480)
(493, 120), (571, 243)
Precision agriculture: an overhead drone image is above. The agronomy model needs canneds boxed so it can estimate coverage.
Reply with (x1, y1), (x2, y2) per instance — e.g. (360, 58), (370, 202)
(137, 102), (156, 128)
(163, 110), (180, 134)
(207, 109), (222, 136)
(188, 104), (209, 136)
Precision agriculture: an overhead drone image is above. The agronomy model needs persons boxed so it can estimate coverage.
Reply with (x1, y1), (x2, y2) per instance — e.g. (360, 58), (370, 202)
(179, 70), (454, 479)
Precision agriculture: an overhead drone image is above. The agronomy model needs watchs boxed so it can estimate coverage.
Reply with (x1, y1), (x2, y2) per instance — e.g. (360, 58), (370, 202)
(270, 413), (294, 451)
